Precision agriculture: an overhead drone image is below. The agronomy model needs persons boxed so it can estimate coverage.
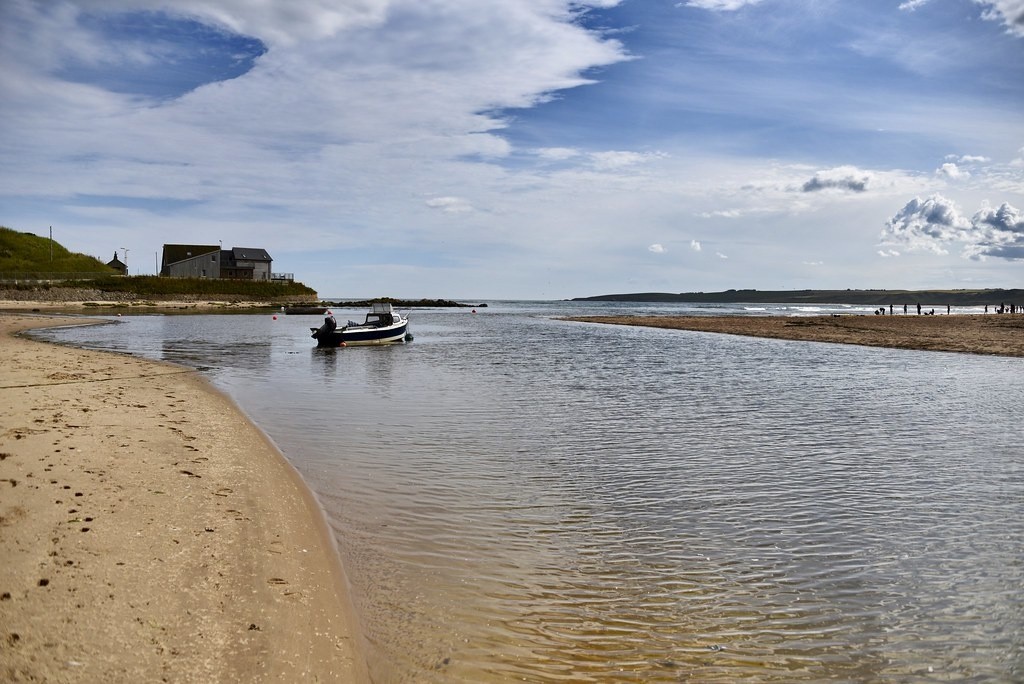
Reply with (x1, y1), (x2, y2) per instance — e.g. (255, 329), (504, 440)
(875, 308), (885, 316)
(904, 304), (907, 315)
(889, 304), (893, 315)
(924, 308), (935, 315)
(917, 302), (921, 315)
(985, 303), (1024, 314)
(947, 303), (950, 316)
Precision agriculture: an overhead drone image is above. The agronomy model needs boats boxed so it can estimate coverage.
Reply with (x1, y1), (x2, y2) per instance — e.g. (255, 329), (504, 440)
(282, 303), (329, 315)
(310, 301), (413, 348)
